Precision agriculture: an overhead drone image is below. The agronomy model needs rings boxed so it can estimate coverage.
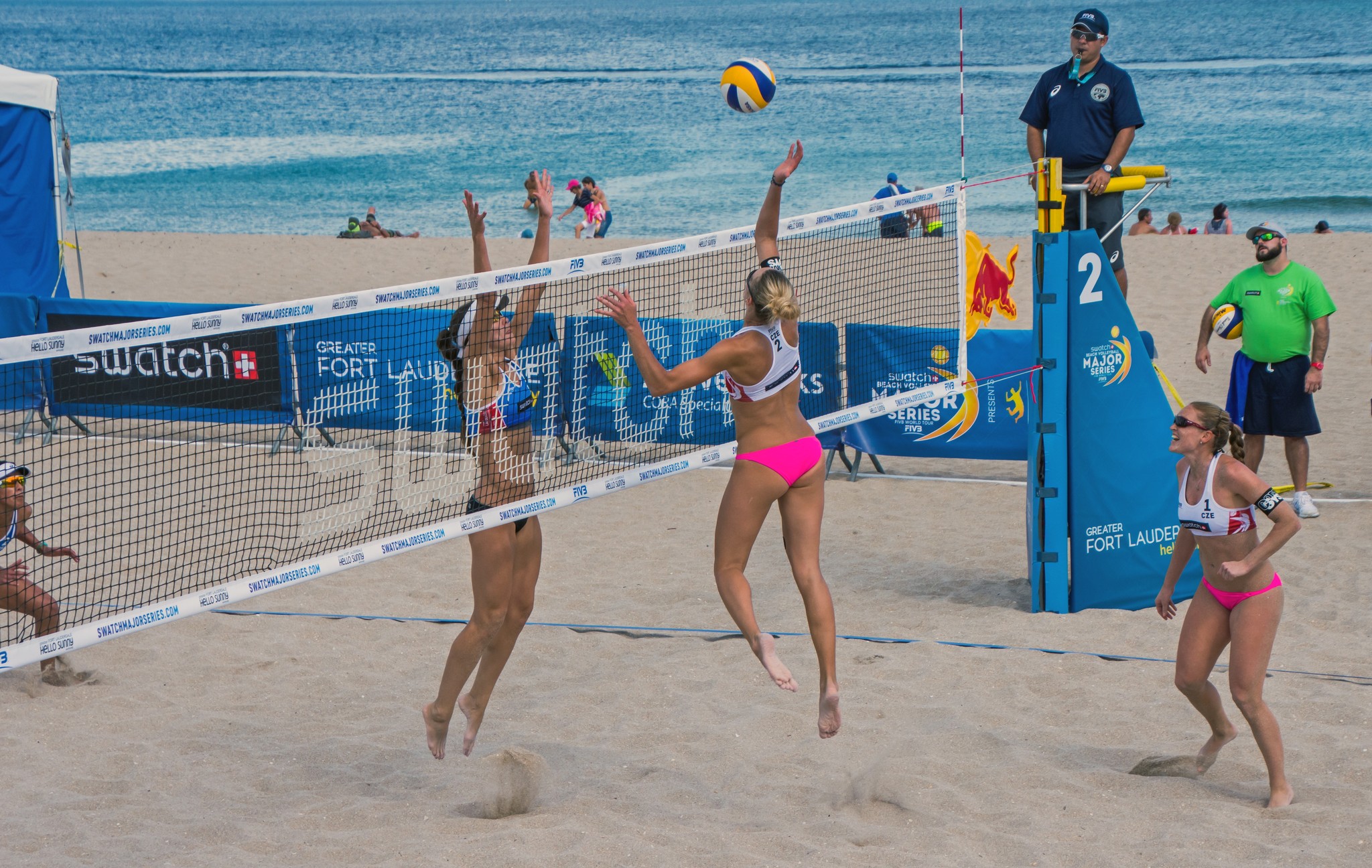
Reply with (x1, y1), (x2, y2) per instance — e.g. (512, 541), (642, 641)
(1100, 185), (1104, 187)
(545, 189), (549, 193)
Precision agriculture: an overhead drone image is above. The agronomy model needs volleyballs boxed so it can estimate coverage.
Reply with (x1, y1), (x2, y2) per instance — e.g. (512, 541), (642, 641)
(721, 57), (775, 114)
(1212, 301), (1242, 340)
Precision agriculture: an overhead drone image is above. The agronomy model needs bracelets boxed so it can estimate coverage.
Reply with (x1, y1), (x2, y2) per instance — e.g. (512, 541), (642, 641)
(771, 175), (786, 187)
(380, 227), (382, 230)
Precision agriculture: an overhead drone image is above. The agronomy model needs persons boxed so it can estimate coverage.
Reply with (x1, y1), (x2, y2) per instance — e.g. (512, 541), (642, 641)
(907, 186), (944, 238)
(1128, 208), (1161, 236)
(1161, 212), (1189, 235)
(522, 171), (541, 209)
(594, 139), (842, 739)
(346, 206), (420, 238)
(582, 176), (612, 239)
(870, 172), (915, 239)
(1018, 8), (1145, 304)
(422, 167), (557, 761)
(559, 179), (600, 239)
(1204, 203), (1233, 234)
(1153, 402), (1301, 812)
(0, 460), (80, 686)
(1312, 220), (1334, 234)
(1195, 220), (1337, 519)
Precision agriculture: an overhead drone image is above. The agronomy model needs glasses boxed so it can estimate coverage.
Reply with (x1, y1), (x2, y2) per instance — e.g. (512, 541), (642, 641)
(1252, 233), (1283, 245)
(0, 475), (26, 488)
(1173, 414), (1207, 430)
(570, 188), (574, 191)
(493, 310), (502, 322)
(1070, 28), (1105, 41)
(746, 268), (757, 300)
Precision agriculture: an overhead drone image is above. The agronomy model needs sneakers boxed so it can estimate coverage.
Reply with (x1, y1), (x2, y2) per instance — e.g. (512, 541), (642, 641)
(1292, 490), (1319, 518)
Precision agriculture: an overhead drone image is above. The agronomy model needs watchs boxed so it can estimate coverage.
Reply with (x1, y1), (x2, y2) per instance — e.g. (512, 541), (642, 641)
(1101, 163), (1113, 176)
(1311, 362), (1324, 370)
(37, 542), (48, 554)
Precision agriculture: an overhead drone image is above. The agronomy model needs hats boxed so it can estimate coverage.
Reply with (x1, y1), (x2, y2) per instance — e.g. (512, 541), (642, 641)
(456, 294), (510, 358)
(0, 462), (31, 479)
(887, 172), (898, 183)
(348, 217), (361, 231)
(566, 179), (579, 190)
(1071, 8), (1109, 35)
(1246, 221), (1287, 239)
